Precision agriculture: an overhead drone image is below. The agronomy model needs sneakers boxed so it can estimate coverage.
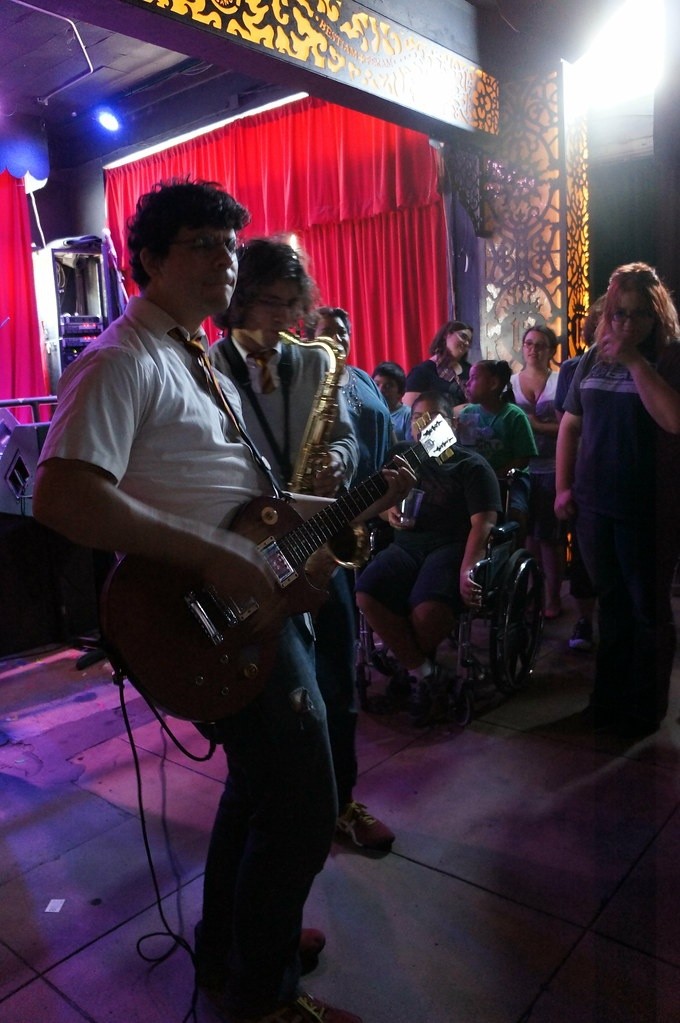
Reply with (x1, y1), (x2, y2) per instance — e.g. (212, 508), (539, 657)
(334, 801), (395, 847)
(215, 991), (362, 1023)
(299, 928), (327, 952)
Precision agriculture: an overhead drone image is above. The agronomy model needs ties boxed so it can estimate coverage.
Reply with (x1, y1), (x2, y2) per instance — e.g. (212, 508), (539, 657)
(175, 329), (240, 433)
(253, 351), (276, 392)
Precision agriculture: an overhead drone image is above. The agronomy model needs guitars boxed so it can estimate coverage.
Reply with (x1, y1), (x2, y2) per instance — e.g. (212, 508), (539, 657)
(105, 414), (458, 726)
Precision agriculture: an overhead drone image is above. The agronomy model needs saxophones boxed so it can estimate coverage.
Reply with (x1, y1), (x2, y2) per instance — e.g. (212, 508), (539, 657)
(276, 326), (373, 614)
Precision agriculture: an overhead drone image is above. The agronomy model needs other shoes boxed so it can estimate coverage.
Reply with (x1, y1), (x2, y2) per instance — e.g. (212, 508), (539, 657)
(570, 617), (594, 647)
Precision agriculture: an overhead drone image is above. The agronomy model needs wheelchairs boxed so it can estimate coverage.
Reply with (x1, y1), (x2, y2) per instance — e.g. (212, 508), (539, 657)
(351, 466), (547, 733)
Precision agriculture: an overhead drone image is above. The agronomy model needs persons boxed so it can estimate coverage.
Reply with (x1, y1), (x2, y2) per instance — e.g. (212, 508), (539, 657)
(31, 172), (417, 1022)
(363, 318), (568, 723)
(209, 234), (397, 853)
(556, 262), (680, 759)
(306, 302), (393, 642)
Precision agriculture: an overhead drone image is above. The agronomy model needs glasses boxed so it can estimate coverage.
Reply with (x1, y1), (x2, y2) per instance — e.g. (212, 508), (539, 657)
(173, 234), (247, 262)
(612, 312), (648, 322)
